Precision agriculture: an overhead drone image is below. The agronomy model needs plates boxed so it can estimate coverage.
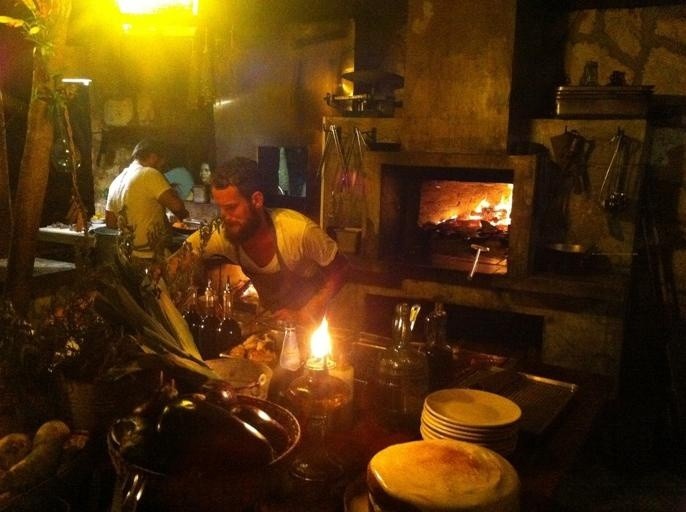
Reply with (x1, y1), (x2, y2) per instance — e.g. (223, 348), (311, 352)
(419, 387), (523, 464)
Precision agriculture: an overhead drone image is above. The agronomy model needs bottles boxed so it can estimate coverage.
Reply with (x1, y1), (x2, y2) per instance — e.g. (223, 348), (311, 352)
(267, 301), (458, 484)
(181, 285), (242, 362)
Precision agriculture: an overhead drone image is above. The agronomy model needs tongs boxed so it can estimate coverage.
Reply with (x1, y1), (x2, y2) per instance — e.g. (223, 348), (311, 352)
(313, 124), (370, 196)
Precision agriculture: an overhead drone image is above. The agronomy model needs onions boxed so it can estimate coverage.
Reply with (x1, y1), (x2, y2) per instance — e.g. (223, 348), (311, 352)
(194, 378), (239, 411)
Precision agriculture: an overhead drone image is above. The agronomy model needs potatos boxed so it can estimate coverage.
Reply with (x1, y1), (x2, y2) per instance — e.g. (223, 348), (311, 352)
(0, 420), (70, 470)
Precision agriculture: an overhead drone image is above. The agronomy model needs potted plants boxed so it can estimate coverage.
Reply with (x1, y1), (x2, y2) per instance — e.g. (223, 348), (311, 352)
(0, 0), (172, 435)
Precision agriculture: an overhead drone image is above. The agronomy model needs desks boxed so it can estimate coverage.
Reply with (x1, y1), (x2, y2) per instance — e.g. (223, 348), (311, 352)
(38, 221), (96, 249)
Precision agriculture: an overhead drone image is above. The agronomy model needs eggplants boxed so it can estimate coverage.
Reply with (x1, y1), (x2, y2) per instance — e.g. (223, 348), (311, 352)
(232, 404), (289, 452)
(157, 395), (273, 472)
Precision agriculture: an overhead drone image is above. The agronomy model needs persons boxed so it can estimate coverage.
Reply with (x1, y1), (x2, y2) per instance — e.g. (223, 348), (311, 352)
(185, 158), (219, 203)
(166, 157), (352, 326)
(163, 155), (195, 200)
(104, 136), (189, 266)
